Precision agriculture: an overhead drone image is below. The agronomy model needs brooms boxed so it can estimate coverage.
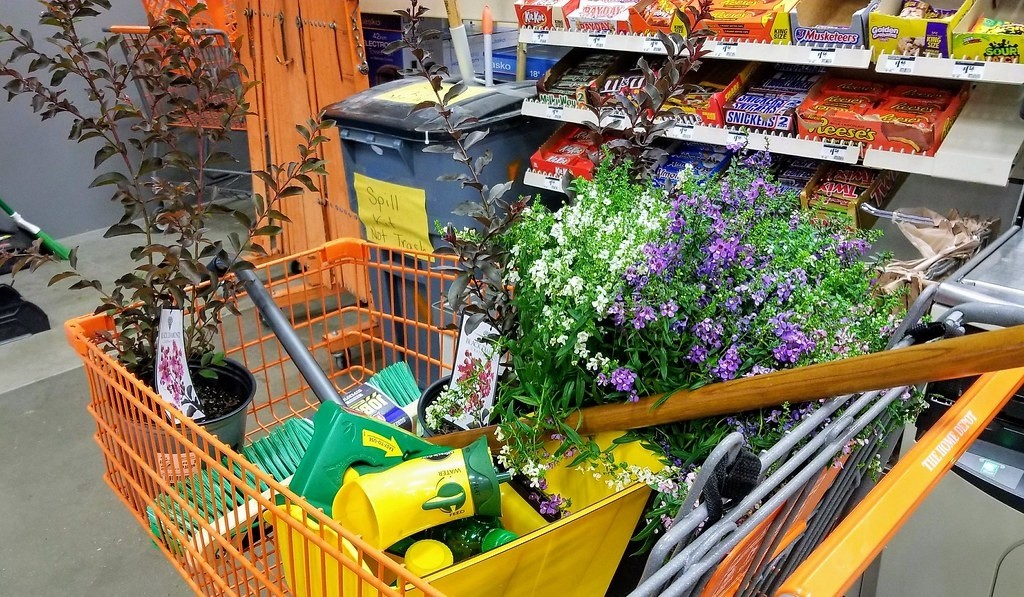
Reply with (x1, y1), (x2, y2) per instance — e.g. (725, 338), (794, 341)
(142, 321), (1023, 576)
(0, 197), (72, 261)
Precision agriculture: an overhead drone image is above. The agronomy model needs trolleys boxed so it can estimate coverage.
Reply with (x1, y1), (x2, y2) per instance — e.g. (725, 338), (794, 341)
(64, 236), (1024, 597)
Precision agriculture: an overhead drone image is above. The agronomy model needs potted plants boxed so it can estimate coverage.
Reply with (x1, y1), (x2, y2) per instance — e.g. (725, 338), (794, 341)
(380, 0), (715, 473)
(0, 0), (336, 499)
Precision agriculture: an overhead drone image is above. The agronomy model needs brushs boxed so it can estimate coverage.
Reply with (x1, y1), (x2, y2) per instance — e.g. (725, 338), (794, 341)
(146, 357), (425, 567)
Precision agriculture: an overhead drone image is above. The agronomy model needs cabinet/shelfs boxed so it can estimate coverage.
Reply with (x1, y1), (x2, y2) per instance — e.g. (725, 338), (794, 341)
(517, 26), (1024, 199)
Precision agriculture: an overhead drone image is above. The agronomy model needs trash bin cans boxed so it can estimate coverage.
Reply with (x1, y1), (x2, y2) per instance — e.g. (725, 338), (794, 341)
(318, 69), (549, 397)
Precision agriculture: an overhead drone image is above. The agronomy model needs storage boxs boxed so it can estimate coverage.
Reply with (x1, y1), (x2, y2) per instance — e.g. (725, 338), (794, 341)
(587, 61), (659, 110)
(868, 0), (974, 63)
(514, 0), (579, 30)
(647, 60), (761, 127)
(789, 0), (876, 49)
(360, 12), (442, 31)
(483, 44), (573, 79)
(363, 27), (444, 87)
(536, 48), (623, 109)
(628, 0), (694, 37)
(445, 23), (518, 74)
(795, 70), (971, 157)
(800, 165), (909, 229)
(721, 62), (799, 138)
(565, 0), (639, 35)
(530, 123), (624, 181)
(951, 0), (1024, 64)
(684, 0), (800, 45)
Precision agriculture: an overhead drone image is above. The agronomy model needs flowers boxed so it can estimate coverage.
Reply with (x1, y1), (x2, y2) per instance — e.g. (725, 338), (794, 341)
(432, 123), (931, 557)
